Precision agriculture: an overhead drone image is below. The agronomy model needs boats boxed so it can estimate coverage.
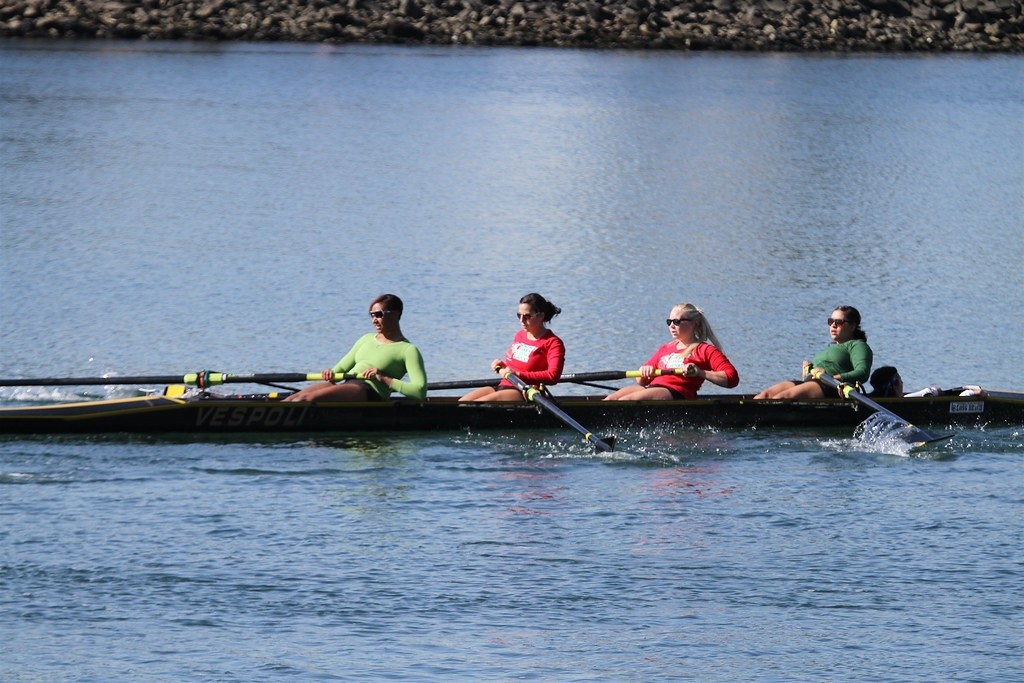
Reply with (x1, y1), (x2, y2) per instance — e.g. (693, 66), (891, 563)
(0, 383), (1024, 443)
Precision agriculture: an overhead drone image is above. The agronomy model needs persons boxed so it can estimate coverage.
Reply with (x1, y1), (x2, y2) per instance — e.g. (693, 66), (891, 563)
(754, 306), (873, 397)
(870, 366), (985, 397)
(602, 303), (739, 400)
(458, 293), (566, 401)
(284, 294), (427, 402)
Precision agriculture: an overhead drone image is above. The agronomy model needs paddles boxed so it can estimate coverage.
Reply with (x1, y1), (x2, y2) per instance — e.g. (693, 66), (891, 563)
(0, 369), (372, 393)
(392, 365), (696, 390)
(495, 364), (613, 454)
(802, 362), (956, 443)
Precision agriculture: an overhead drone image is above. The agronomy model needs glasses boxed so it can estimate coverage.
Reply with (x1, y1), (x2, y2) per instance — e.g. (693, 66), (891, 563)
(828, 318), (851, 326)
(517, 313), (540, 320)
(369, 310), (395, 318)
(667, 318), (691, 326)
